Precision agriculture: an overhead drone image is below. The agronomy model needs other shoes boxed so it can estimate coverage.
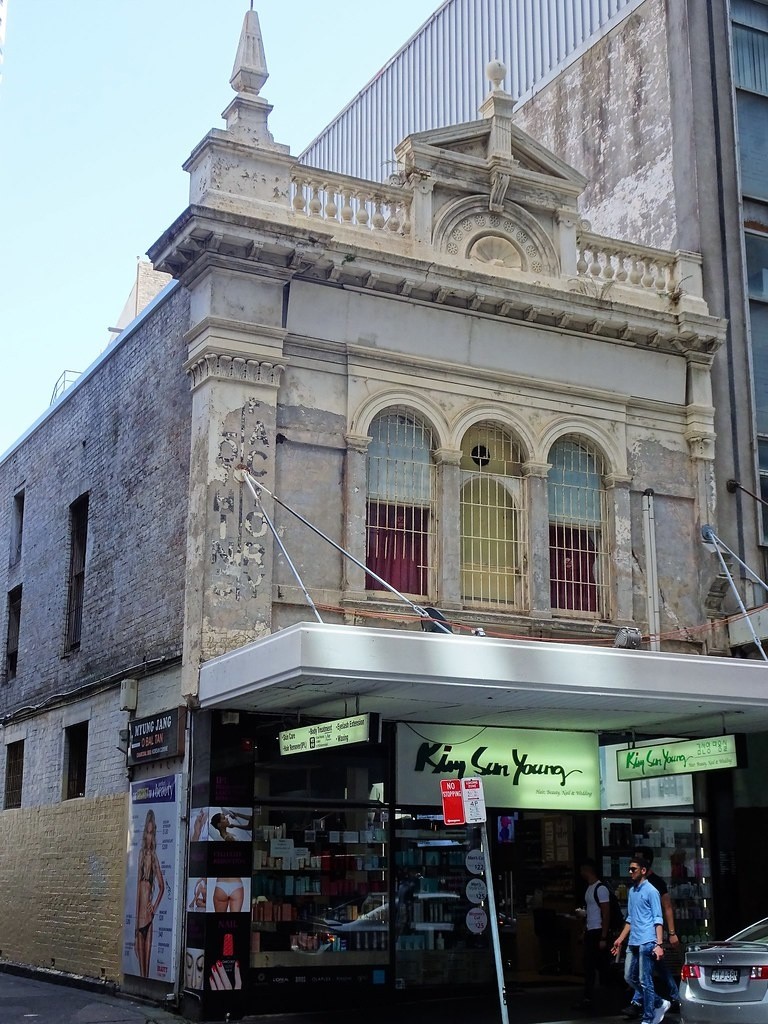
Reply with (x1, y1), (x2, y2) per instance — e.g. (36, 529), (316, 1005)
(668, 1002), (681, 1014)
(621, 1005), (639, 1019)
(575, 1002), (593, 1010)
(652, 999), (671, 1024)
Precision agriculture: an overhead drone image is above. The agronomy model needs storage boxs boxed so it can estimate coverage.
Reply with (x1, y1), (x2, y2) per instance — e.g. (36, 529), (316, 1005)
(283, 856), (290, 870)
(258, 901), (272, 921)
(254, 850), (261, 869)
(329, 831), (339, 843)
(352, 906), (358, 920)
(282, 904), (291, 921)
(343, 832), (358, 843)
(424, 927), (434, 949)
(347, 906), (352, 920)
(285, 876), (293, 895)
(250, 932), (259, 952)
(360, 831), (373, 843)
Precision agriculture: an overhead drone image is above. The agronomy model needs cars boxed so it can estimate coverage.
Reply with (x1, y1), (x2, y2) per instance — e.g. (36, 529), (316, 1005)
(678, 918), (768, 1024)
(289, 892), (516, 955)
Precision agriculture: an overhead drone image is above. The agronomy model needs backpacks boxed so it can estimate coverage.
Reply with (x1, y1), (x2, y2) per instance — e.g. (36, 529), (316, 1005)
(594, 880), (625, 929)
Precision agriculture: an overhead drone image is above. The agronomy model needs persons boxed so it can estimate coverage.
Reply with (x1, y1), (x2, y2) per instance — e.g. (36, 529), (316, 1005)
(610, 857), (671, 1024)
(191, 809), (252, 841)
(134, 810), (164, 978)
(621, 846), (679, 1018)
(574, 859), (611, 1010)
(185, 948), (242, 990)
(189, 878), (245, 912)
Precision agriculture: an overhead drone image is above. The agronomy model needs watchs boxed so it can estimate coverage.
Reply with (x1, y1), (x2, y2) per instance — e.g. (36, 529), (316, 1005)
(657, 944), (664, 948)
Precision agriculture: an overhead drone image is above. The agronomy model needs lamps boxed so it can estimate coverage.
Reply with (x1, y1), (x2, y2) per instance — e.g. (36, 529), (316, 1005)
(613, 626), (642, 650)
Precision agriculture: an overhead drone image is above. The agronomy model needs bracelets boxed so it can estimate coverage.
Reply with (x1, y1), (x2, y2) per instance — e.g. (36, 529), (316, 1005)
(599, 936), (608, 941)
(669, 929), (675, 936)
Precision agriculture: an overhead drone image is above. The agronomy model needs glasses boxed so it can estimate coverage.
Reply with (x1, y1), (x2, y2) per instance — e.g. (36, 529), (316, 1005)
(629, 867), (640, 872)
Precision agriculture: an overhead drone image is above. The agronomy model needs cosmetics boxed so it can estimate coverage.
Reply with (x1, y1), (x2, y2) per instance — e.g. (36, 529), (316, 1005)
(251, 823), (387, 953)
(396, 848), (475, 950)
(634, 830), (711, 943)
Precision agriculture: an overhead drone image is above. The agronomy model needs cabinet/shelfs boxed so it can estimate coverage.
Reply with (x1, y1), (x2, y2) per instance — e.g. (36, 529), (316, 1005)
(250, 805), (709, 967)
